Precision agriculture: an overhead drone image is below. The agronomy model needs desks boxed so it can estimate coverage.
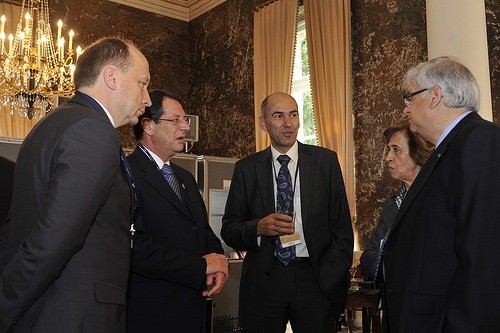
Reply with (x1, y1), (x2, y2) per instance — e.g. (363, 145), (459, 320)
(343, 288), (383, 333)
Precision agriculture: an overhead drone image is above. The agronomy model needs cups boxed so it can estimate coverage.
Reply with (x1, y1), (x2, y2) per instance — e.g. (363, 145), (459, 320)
(278, 210), (296, 229)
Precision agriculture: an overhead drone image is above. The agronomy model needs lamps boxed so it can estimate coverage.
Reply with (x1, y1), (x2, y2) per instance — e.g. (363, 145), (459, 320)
(0, 0), (84, 120)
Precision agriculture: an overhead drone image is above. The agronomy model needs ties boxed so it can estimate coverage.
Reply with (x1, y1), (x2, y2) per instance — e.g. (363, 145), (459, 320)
(160, 163), (182, 201)
(276, 154), (296, 266)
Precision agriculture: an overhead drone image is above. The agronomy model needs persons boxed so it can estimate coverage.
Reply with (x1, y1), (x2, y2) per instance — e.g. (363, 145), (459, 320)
(125, 90), (229, 333)
(0, 38), (153, 333)
(220, 92), (354, 333)
(375, 54), (500, 332)
(357, 122), (433, 333)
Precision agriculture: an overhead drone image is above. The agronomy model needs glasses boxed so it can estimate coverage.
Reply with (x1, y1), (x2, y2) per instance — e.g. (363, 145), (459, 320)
(403, 88), (428, 103)
(160, 116), (191, 125)
